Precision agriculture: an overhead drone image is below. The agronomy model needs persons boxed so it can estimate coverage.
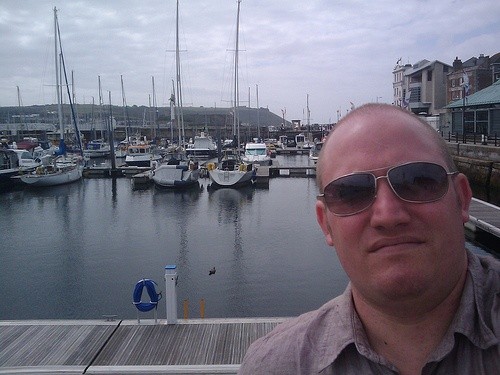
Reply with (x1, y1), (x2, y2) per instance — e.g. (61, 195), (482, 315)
(236, 103), (500, 375)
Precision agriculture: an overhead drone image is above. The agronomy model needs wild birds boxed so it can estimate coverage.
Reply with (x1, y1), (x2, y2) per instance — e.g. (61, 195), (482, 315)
(209, 266), (216, 275)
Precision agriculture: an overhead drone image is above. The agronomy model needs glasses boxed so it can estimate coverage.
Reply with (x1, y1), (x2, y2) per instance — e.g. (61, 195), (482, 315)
(316, 161), (460, 217)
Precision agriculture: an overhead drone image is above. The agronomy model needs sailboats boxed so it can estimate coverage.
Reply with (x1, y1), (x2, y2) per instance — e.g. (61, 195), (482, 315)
(1, 0), (324, 188)
